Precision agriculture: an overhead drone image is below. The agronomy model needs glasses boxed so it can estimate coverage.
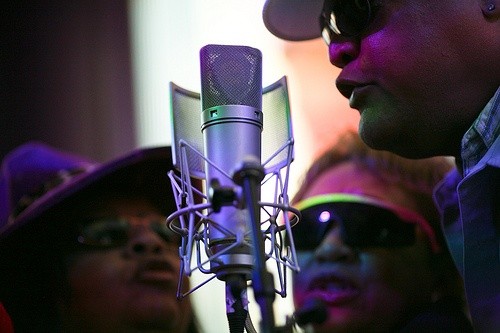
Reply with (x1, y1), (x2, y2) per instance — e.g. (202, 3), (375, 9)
(70, 218), (195, 250)
(319, 0), (386, 46)
(285, 205), (433, 251)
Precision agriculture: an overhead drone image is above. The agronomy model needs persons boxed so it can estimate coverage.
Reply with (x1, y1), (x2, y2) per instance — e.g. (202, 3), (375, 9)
(261, 0), (500, 333)
(0, 142), (206, 333)
(281, 128), (470, 333)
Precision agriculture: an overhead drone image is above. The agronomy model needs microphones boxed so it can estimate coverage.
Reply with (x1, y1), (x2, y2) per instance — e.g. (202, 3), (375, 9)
(199, 43), (265, 333)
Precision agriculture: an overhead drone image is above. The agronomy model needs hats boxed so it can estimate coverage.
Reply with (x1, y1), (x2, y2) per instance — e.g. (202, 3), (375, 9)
(1, 142), (204, 237)
(282, 158), (443, 254)
(262, 0), (325, 41)
